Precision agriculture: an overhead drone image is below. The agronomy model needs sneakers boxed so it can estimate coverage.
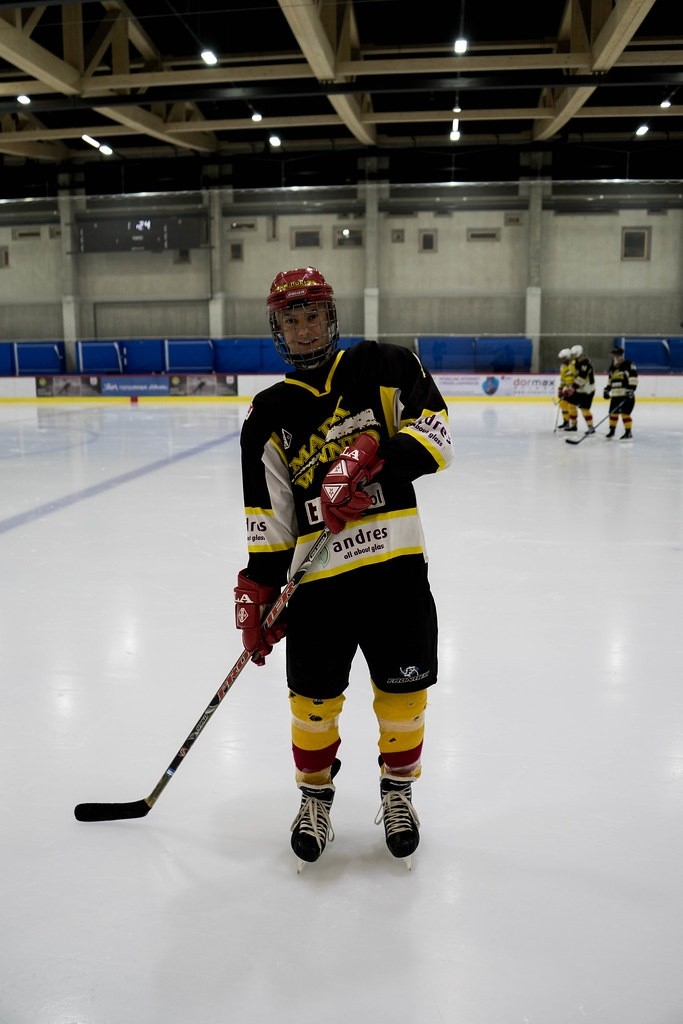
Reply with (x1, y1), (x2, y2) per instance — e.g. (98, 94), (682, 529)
(374, 755), (421, 872)
(289, 757), (341, 874)
(605, 429), (614, 440)
(619, 431), (633, 441)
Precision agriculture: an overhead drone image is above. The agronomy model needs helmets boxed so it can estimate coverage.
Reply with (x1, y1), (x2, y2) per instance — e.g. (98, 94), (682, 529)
(558, 348), (571, 361)
(572, 345), (583, 357)
(266, 266), (339, 369)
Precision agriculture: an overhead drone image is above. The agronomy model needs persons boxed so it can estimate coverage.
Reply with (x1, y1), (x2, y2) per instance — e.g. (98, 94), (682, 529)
(603, 347), (638, 442)
(233, 267), (455, 874)
(555, 345), (595, 435)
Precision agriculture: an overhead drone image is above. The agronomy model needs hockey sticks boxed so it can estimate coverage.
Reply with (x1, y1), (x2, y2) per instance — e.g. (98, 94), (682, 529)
(74, 525), (331, 823)
(565, 399), (628, 445)
(551, 391), (569, 434)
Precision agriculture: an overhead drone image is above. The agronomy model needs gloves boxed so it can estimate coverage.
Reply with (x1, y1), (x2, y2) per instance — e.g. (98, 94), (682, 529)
(603, 389), (610, 400)
(564, 387), (574, 397)
(321, 433), (384, 535)
(233, 568), (286, 667)
(624, 390), (633, 399)
(558, 388), (563, 397)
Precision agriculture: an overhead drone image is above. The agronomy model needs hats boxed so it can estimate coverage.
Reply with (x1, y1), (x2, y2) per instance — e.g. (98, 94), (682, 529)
(610, 347), (623, 356)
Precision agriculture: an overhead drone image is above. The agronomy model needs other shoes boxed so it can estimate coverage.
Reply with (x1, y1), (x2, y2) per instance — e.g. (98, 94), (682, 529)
(585, 429), (595, 434)
(558, 424), (569, 428)
(564, 427), (577, 431)
